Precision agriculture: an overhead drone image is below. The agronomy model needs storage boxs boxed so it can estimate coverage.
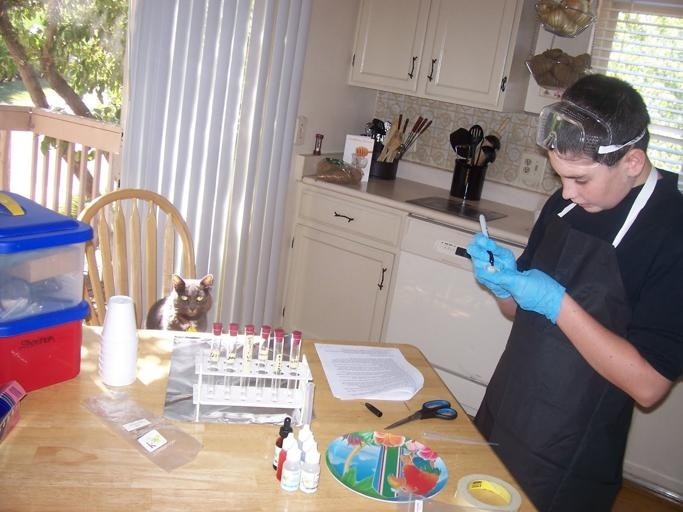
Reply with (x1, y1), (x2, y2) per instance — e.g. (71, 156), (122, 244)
(0, 314), (83, 391)
(0, 188), (93, 323)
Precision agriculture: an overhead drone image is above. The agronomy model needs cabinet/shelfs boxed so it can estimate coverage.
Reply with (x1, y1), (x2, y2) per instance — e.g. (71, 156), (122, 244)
(347, 0), (528, 114)
(283, 173), (409, 349)
(380, 209), (683, 506)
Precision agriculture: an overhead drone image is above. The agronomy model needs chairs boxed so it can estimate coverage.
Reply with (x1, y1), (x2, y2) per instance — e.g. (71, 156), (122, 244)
(78, 189), (195, 328)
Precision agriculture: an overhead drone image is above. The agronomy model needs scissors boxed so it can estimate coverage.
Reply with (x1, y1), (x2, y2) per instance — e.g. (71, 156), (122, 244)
(384, 400), (457, 429)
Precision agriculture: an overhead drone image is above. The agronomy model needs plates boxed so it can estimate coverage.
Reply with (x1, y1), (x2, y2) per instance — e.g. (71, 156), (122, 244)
(324, 430), (450, 502)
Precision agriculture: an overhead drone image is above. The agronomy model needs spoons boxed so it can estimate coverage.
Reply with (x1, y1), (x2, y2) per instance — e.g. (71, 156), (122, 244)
(481, 144), (495, 165)
(484, 134), (502, 151)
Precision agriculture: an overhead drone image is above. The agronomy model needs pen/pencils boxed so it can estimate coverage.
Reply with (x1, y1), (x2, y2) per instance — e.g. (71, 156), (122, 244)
(479, 214), (495, 267)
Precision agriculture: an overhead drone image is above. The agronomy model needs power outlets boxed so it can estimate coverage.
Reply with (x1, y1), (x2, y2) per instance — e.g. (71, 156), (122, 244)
(518, 154), (546, 186)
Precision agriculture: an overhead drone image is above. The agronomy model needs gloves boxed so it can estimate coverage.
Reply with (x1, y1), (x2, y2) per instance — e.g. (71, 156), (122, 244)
(479, 268), (567, 326)
(464, 233), (518, 299)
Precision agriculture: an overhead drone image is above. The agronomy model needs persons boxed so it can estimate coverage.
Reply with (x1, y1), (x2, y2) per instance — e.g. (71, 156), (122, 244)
(465, 73), (683, 512)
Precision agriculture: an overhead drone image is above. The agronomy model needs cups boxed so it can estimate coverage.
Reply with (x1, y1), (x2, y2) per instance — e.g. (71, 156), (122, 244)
(448, 158), (490, 202)
(97, 295), (137, 387)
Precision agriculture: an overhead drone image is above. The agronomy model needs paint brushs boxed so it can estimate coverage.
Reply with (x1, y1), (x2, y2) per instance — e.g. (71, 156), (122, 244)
(364, 402), (383, 418)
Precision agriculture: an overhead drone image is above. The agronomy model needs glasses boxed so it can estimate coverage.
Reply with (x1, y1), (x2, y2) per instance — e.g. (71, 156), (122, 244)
(534, 101), (650, 167)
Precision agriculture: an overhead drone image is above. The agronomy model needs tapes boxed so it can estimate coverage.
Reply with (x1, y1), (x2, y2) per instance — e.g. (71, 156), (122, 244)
(456, 474), (521, 512)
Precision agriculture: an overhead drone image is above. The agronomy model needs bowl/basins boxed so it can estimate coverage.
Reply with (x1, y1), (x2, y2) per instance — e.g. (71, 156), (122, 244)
(524, 59), (599, 92)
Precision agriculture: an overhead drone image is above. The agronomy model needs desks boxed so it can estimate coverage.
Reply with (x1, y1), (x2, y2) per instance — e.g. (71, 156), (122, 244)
(0, 326), (542, 512)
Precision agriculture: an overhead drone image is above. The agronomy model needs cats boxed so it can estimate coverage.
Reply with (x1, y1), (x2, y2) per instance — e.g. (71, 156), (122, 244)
(144, 272), (213, 332)
